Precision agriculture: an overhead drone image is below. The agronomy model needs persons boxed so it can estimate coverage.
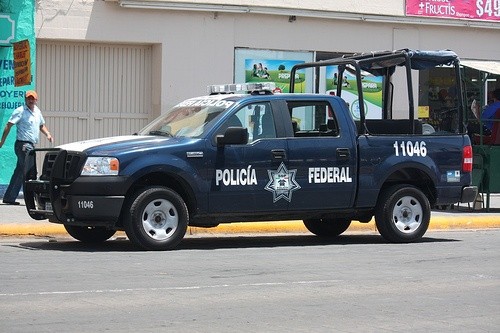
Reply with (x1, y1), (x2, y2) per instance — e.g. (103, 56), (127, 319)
(482, 89), (500, 135)
(344, 76), (349, 87)
(251, 63), (269, 79)
(0, 91), (54, 204)
(332, 72), (338, 84)
(438, 87), (458, 131)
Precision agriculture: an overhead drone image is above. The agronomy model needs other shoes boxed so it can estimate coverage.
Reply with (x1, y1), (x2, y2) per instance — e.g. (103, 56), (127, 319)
(3, 198), (20, 205)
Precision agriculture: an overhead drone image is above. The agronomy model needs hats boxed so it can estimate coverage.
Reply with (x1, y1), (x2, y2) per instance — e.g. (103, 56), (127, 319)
(25, 90), (38, 100)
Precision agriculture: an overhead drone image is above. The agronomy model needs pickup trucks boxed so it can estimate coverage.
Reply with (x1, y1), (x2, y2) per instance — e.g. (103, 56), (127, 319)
(24, 47), (477, 250)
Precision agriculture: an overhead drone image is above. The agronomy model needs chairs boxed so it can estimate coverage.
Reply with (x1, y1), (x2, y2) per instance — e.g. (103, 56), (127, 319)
(257, 115), (276, 139)
(457, 152), (485, 210)
